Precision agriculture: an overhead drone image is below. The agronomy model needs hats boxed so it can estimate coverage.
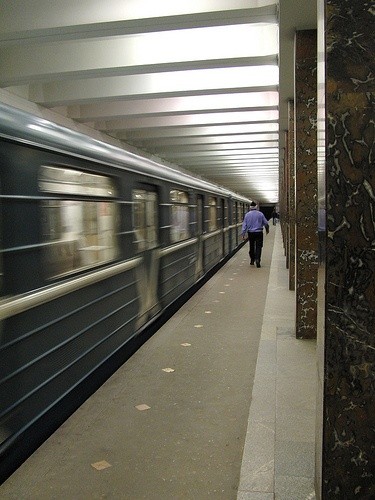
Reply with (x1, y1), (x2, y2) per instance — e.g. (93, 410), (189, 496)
(250, 202), (257, 206)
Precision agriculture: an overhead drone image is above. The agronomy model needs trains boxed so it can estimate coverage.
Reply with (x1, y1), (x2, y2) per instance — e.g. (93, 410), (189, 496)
(0, 103), (259, 476)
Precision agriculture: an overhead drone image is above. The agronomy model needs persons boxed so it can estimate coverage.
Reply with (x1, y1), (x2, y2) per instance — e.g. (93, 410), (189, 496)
(277, 212), (280, 219)
(271, 208), (277, 225)
(240, 201), (269, 268)
